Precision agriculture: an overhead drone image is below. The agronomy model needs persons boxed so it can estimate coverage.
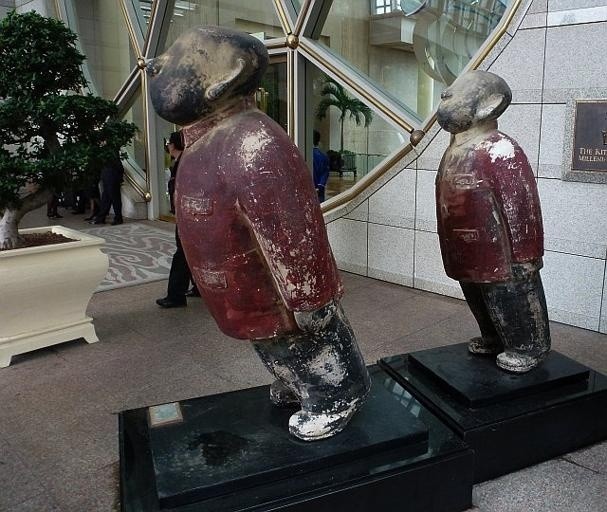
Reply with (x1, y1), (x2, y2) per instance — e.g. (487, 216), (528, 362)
(311, 130), (330, 204)
(431, 68), (552, 375)
(43, 134), (124, 226)
(155, 131), (201, 310)
(143, 24), (374, 444)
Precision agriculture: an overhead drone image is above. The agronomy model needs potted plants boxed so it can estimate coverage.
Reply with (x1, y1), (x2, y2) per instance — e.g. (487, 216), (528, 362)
(314, 74), (376, 179)
(0, 10), (137, 367)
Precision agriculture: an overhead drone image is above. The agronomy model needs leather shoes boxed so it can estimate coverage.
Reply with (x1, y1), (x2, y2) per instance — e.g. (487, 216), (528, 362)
(187, 288), (201, 296)
(71, 211), (123, 225)
(156, 295), (187, 307)
(49, 215), (64, 218)
